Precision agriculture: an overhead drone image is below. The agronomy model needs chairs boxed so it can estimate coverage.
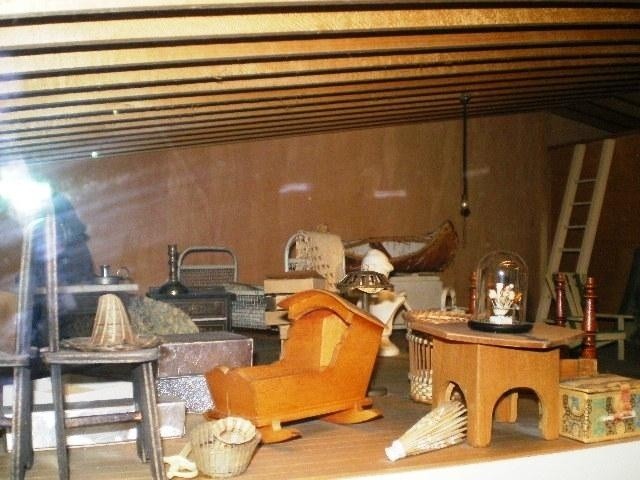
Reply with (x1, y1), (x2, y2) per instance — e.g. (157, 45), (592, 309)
(543, 269), (633, 362)
(46, 204), (169, 479)
(0, 218), (46, 480)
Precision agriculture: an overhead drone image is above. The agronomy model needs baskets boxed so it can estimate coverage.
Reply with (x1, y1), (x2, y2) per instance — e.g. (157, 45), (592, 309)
(402, 309), (472, 404)
(190, 420), (261, 478)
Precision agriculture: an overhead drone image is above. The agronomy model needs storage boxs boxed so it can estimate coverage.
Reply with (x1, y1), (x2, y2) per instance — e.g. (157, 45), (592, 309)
(553, 372), (640, 443)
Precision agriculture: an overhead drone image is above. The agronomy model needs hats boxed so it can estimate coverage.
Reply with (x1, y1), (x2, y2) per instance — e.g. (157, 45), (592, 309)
(60, 293), (160, 352)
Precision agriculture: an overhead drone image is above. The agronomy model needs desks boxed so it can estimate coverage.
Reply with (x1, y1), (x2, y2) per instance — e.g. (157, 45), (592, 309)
(405, 321), (585, 448)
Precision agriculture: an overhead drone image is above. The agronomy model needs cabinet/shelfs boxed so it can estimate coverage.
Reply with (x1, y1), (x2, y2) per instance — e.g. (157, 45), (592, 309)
(147, 284), (236, 333)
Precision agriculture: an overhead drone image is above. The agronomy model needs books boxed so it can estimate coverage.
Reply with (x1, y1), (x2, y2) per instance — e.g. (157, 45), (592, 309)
(259, 267), (329, 360)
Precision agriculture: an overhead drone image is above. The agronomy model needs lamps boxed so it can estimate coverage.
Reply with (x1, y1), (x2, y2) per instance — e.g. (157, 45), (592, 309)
(337, 270), (394, 397)
